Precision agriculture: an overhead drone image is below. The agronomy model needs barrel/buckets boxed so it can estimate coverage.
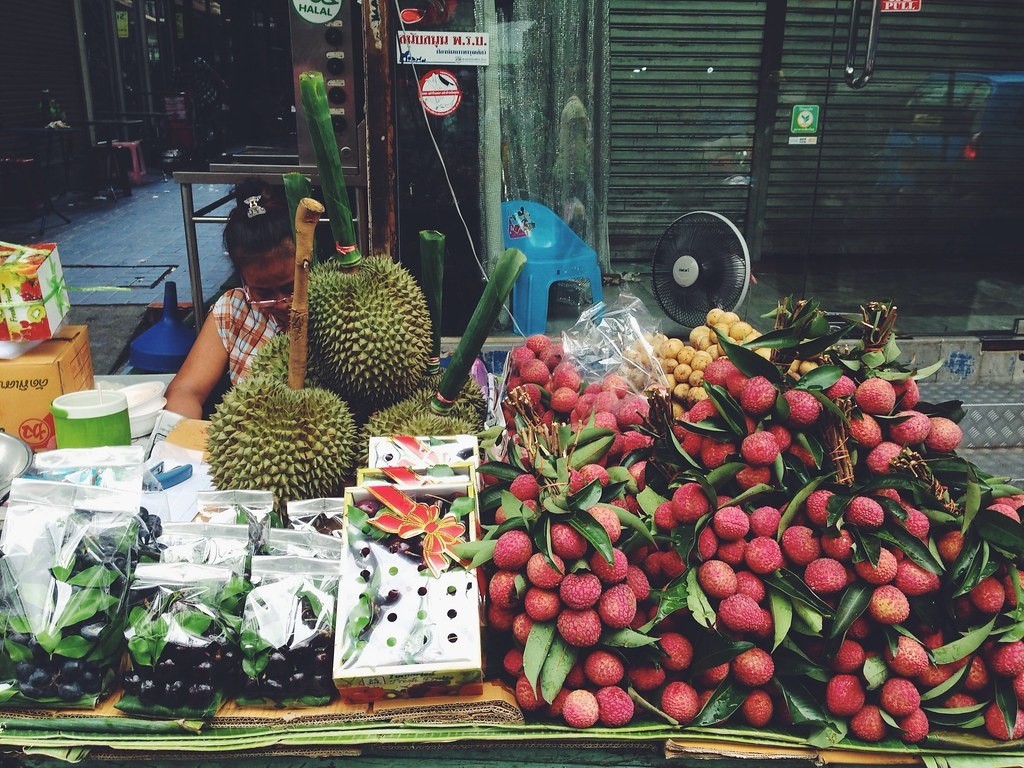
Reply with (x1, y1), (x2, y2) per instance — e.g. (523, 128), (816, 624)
(49, 388), (132, 449)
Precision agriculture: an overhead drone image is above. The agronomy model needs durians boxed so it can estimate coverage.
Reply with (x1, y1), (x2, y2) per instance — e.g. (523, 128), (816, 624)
(200, 249), (492, 513)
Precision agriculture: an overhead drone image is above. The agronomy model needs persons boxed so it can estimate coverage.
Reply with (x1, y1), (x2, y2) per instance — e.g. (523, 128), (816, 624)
(161, 176), (293, 419)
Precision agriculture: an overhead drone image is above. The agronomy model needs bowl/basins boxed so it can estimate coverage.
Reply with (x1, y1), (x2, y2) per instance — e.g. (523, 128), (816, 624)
(129, 396), (168, 439)
(118, 381), (165, 415)
(0, 427), (33, 508)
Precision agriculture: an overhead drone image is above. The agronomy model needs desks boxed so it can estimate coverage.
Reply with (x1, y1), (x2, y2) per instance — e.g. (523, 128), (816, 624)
(48, 119), (142, 203)
(96, 111), (180, 182)
(1, 126), (86, 236)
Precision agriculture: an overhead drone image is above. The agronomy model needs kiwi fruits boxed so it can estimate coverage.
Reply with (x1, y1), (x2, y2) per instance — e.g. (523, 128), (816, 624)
(26, 305), (45, 323)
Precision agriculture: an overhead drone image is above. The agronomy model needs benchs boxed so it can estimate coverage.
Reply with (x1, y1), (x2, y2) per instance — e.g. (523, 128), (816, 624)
(90, 141), (145, 184)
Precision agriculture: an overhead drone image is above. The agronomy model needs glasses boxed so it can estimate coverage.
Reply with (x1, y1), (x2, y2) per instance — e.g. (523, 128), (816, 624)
(239, 270), (297, 314)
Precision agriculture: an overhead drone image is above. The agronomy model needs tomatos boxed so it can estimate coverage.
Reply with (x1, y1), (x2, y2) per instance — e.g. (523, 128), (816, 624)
(20, 277), (42, 298)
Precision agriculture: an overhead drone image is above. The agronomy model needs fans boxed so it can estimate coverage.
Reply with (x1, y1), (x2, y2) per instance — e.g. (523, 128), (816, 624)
(651, 211), (751, 330)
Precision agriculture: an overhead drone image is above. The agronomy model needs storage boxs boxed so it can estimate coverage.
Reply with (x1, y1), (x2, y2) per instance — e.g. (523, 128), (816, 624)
(333, 434), (487, 704)
(0, 325), (94, 453)
(0, 243), (69, 341)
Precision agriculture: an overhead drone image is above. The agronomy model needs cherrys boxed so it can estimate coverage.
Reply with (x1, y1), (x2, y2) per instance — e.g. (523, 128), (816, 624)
(347, 493), (473, 568)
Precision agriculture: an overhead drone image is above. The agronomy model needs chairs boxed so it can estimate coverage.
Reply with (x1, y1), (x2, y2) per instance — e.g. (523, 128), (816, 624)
(500, 200), (604, 339)
